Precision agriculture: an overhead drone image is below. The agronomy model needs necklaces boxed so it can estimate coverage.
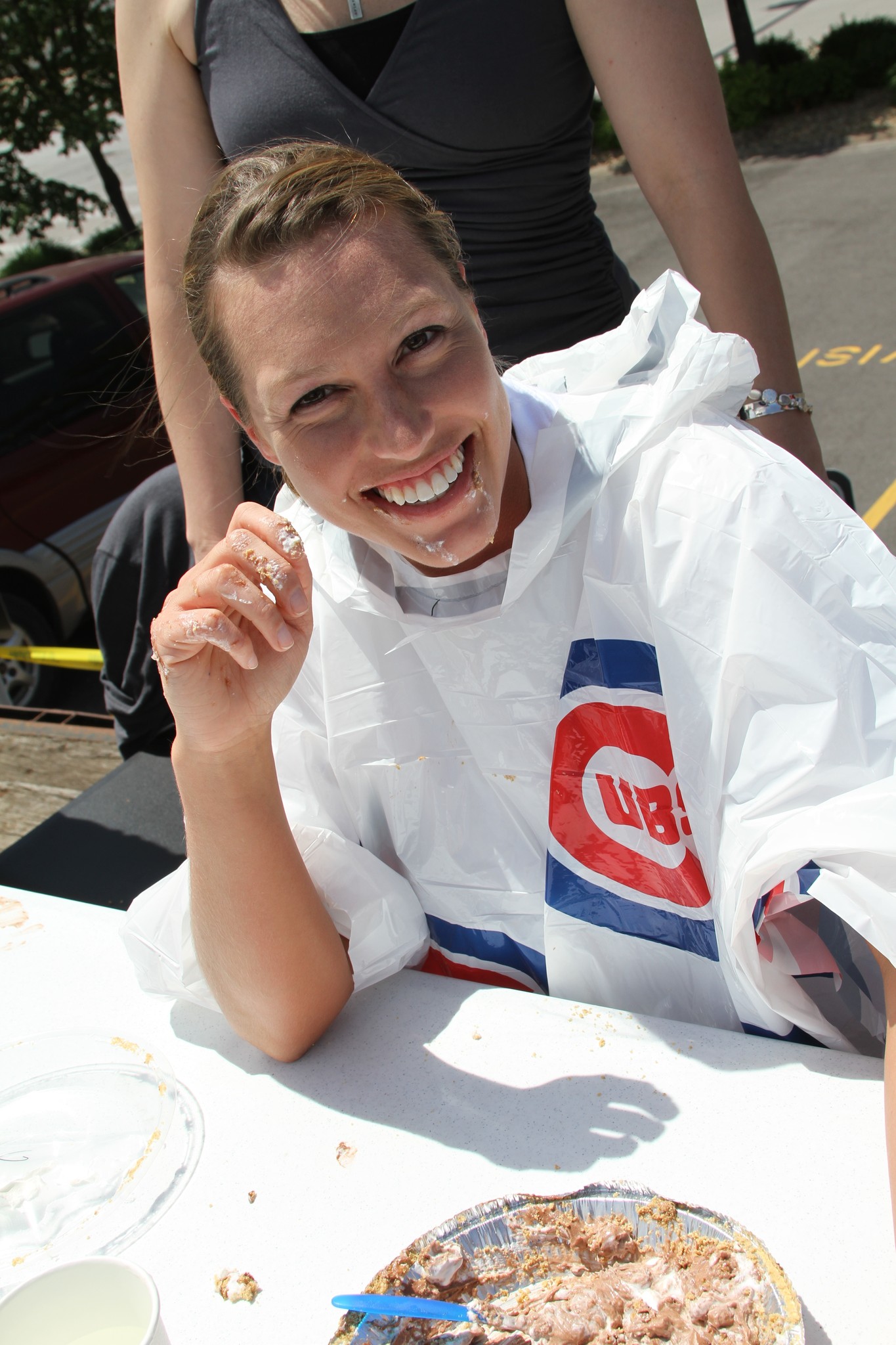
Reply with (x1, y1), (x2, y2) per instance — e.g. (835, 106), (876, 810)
(346, 0), (363, 19)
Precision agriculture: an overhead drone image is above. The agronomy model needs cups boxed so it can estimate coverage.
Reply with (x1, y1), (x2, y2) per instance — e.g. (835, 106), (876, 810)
(0, 1255), (172, 1345)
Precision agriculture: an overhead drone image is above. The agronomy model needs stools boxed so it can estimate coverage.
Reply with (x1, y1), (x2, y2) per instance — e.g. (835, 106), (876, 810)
(0, 751), (188, 908)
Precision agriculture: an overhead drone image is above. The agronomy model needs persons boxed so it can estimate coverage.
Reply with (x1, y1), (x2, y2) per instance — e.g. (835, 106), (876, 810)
(91, 0), (838, 760)
(125, 138), (896, 1250)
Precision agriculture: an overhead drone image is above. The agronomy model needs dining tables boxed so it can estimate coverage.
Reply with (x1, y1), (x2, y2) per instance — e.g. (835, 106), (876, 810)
(0, 880), (896, 1345)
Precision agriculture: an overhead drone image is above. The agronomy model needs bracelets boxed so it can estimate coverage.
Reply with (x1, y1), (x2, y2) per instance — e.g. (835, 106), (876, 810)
(739, 388), (813, 421)
